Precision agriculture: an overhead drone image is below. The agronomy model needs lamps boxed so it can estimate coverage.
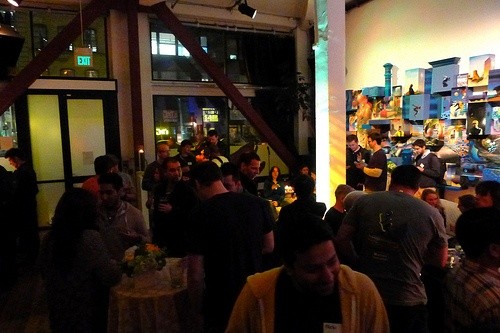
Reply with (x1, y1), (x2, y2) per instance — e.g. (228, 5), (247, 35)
(226, 0), (257, 19)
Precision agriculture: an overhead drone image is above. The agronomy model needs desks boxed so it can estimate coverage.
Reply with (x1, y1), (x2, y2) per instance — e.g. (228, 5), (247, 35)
(108, 258), (187, 333)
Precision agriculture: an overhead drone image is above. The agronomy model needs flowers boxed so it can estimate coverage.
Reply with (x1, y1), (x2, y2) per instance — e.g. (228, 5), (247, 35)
(119, 243), (167, 279)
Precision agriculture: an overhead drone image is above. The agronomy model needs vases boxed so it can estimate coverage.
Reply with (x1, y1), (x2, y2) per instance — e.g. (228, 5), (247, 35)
(128, 270), (162, 291)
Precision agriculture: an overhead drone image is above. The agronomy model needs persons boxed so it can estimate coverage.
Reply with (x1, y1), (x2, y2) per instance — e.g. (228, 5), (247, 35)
(0, 130), (500, 333)
(354, 134), (388, 192)
(227, 214), (391, 333)
(343, 165), (451, 333)
